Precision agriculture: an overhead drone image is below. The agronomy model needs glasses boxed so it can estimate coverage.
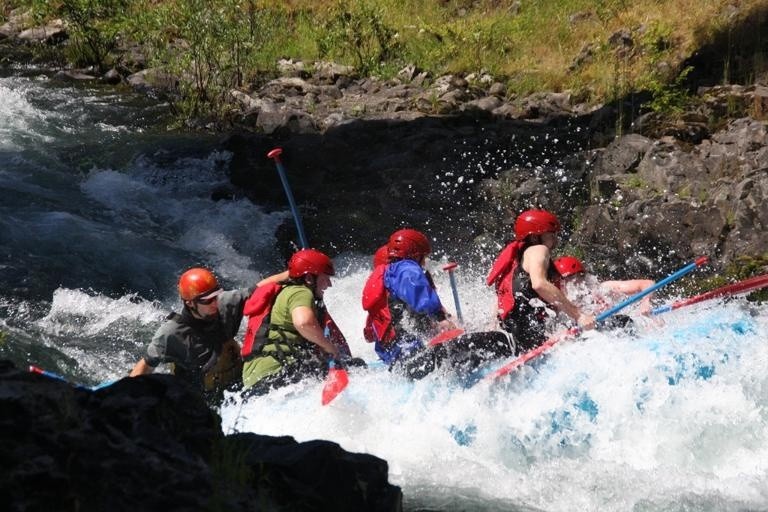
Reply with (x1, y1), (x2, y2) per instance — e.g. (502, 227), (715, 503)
(195, 296), (217, 305)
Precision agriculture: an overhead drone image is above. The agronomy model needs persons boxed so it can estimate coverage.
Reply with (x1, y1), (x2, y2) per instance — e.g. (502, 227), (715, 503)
(242, 250), (368, 403)
(545, 257), (656, 344)
(363, 230), (513, 388)
(486, 209), (597, 358)
(127, 266), (290, 415)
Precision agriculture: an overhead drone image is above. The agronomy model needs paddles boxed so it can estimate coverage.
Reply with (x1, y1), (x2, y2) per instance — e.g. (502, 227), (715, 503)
(427, 308), (464, 348)
(648, 272), (767, 316)
(492, 256), (710, 377)
(321, 310), (349, 406)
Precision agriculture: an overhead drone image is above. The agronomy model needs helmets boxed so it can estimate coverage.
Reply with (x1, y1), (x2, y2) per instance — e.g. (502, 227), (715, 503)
(287, 250), (335, 278)
(514, 210), (561, 242)
(373, 230), (431, 267)
(553, 256), (587, 279)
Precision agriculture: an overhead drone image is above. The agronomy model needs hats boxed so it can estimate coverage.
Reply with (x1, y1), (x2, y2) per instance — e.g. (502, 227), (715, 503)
(179, 268), (224, 300)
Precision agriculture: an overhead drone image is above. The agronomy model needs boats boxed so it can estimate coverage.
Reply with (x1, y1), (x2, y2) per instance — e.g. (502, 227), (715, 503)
(97, 312), (757, 446)
